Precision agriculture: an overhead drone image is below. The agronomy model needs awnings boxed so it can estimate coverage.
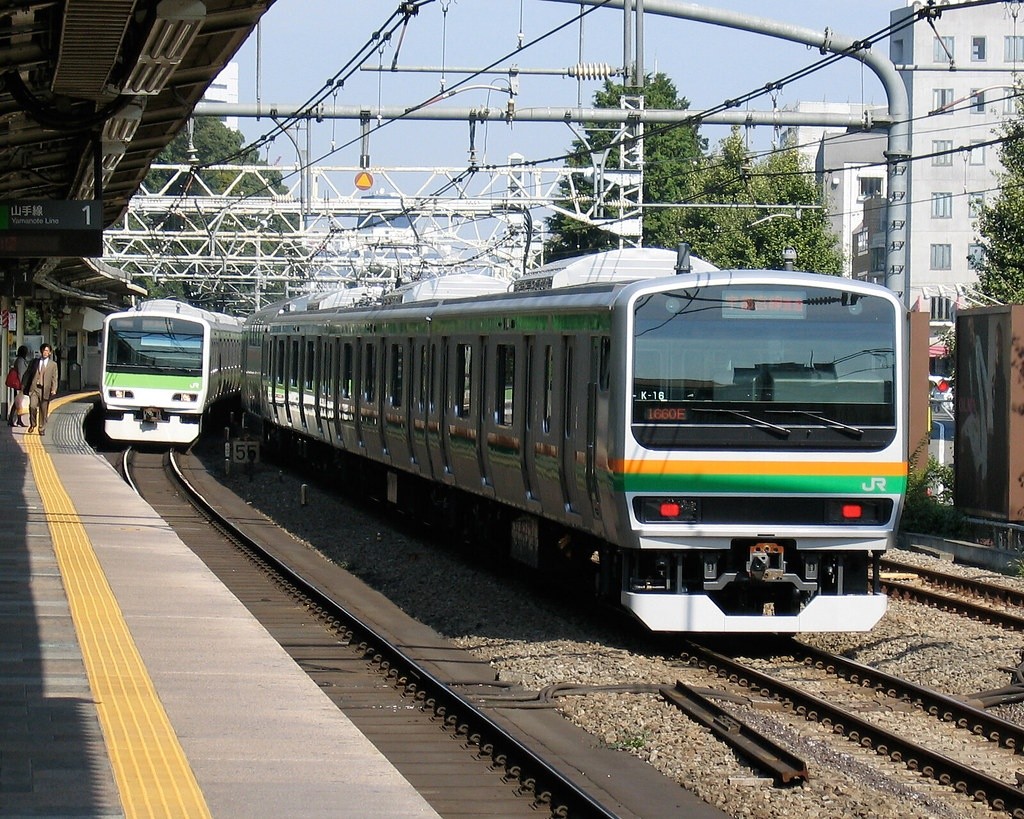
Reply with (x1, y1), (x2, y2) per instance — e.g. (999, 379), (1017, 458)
(929, 336), (953, 358)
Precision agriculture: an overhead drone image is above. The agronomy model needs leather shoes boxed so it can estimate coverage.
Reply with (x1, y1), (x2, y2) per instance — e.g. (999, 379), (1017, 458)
(28, 424), (37, 433)
(39, 431), (44, 436)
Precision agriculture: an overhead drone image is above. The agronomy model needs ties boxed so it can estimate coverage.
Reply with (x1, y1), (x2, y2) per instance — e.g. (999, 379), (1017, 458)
(39, 359), (44, 385)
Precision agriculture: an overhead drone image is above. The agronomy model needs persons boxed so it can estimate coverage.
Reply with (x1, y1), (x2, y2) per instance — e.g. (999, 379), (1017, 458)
(20, 343), (58, 436)
(7, 345), (29, 427)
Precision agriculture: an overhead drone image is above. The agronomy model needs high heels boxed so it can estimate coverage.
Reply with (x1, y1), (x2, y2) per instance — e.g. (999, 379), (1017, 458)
(7, 420), (17, 427)
(16, 419), (28, 427)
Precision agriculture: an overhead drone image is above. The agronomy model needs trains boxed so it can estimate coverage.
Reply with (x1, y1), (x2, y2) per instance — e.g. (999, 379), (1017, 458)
(239, 238), (909, 635)
(98, 296), (248, 445)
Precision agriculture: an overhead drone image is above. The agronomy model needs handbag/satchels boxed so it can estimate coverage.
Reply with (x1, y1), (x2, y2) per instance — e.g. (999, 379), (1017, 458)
(15, 390), (30, 415)
(4, 368), (22, 390)
